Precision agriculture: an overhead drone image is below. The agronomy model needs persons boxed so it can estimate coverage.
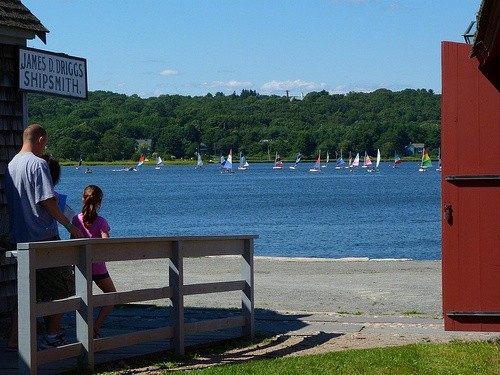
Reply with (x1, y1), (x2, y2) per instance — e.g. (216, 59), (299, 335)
(2, 125), (86, 354)
(39, 154), (67, 216)
(68, 184), (119, 340)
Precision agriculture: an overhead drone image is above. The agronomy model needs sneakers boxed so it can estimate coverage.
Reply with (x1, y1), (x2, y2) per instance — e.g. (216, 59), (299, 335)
(39, 334), (70, 351)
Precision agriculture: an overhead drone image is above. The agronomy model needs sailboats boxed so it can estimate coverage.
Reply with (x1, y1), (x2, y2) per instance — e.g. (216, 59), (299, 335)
(155, 157), (163, 169)
(323, 152), (329, 168)
(273, 151), (283, 169)
(219, 149), (249, 174)
(335, 149), (381, 173)
(289, 152), (301, 170)
(121, 153), (144, 172)
(196, 153), (203, 169)
(419, 148), (433, 171)
(310, 151), (322, 172)
(392, 150), (401, 169)
(436, 148), (442, 172)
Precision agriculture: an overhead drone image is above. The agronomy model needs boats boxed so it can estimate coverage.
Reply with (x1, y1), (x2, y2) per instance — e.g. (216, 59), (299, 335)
(85, 168), (92, 173)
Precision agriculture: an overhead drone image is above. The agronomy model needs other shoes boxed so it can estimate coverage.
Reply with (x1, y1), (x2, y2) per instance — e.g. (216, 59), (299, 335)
(94, 330), (102, 339)
(7, 346), (16, 352)
